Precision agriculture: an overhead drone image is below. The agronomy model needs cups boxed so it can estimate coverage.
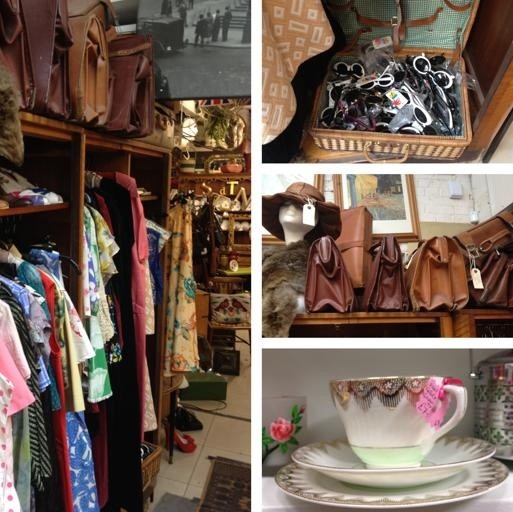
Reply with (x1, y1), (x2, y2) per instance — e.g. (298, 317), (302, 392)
(261, 394), (305, 477)
(177, 157), (196, 175)
(329, 376), (468, 468)
(219, 255), (227, 271)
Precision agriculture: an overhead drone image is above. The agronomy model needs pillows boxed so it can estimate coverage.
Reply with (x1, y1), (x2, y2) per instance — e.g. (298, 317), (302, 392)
(207, 288), (251, 324)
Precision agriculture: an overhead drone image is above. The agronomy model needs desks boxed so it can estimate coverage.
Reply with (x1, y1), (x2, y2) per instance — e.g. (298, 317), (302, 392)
(294, 82), (399, 163)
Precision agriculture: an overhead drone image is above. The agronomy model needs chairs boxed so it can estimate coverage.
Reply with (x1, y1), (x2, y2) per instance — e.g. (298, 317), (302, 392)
(207, 274), (251, 369)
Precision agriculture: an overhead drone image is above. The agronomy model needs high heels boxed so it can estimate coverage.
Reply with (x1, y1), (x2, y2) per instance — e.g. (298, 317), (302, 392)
(166, 423), (196, 453)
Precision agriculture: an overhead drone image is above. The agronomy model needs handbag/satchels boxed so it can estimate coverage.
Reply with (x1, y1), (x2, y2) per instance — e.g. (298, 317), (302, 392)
(470, 229), (510, 309)
(362, 235), (408, 311)
(0, 1), (155, 140)
(303, 237), (354, 313)
(402, 237), (472, 311)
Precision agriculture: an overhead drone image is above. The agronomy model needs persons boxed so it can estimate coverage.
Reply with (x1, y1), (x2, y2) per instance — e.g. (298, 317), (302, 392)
(192, 6), (232, 48)
(178, 4), (189, 29)
(261, 182), (342, 339)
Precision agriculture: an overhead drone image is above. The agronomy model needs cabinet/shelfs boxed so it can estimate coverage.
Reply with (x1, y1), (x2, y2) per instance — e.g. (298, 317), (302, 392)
(193, 286), (210, 345)
(452, 302), (512, 338)
(176, 171), (250, 266)
(283, 307), (453, 337)
(79, 118), (176, 511)
(0, 107), (89, 347)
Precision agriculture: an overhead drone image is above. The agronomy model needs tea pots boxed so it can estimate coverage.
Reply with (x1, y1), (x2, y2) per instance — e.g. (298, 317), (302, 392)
(468, 349), (513, 464)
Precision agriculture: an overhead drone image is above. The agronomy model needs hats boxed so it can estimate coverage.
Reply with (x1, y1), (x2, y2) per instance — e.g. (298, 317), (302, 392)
(262, 182), (343, 245)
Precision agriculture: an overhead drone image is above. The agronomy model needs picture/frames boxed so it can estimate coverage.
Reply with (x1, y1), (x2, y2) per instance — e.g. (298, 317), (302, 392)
(331, 174), (425, 246)
(262, 174), (325, 250)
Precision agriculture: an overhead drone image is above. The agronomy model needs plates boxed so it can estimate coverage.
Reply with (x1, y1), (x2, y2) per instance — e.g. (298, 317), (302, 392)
(274, 457), (511, 511)
(290, 437), (495, 485)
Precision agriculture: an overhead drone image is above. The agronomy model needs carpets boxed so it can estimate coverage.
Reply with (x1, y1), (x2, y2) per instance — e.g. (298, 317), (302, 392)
(194, 453), (250, 512)
